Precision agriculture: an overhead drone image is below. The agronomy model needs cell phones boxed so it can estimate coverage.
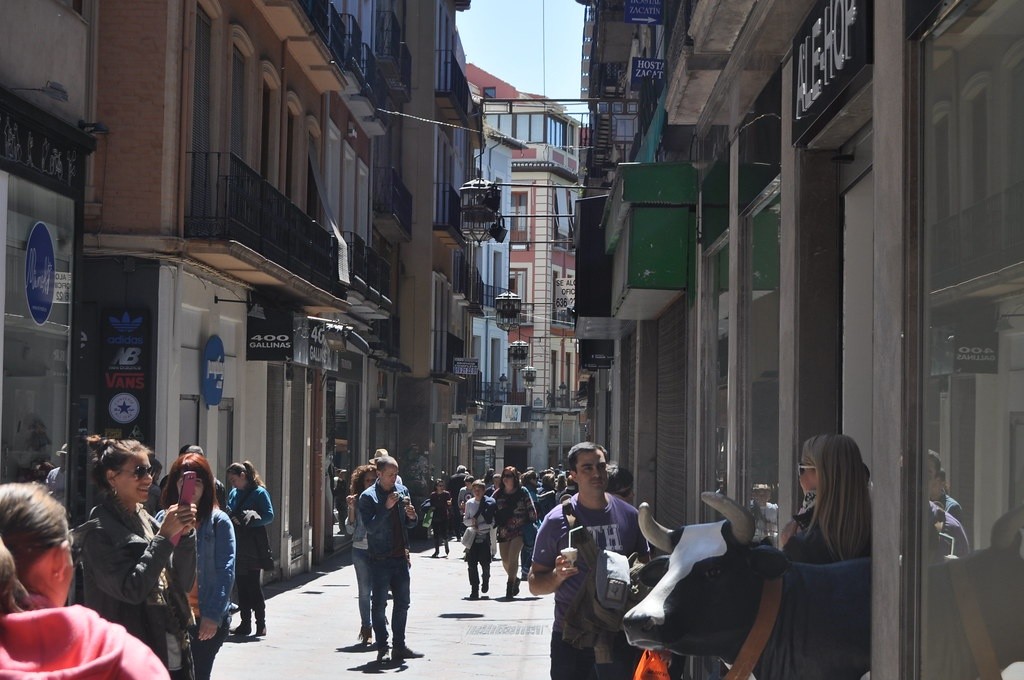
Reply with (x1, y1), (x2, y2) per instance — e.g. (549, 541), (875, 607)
(178, 471), (196, 506)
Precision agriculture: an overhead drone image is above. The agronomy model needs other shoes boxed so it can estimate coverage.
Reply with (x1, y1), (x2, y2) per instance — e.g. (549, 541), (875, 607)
(521, 575), (527, 581)
(391, 646), (424, 659)
(445, 546), (449, 554)
(256, 624), (266, 636)
(229, 621), (251, 635)
(432, 551), (439, 557)
(377, 649), (390, 663)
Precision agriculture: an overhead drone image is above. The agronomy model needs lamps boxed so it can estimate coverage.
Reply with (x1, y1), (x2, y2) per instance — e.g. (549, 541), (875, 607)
(495, 291), (555, 334)
(79, 119), (109, 134)
(10, 80), (69, 103)
(508, 337), (575, 370)
(456, 175), (611, 248)
(215, 296), (266, 320)
(522, 364), (540, 388)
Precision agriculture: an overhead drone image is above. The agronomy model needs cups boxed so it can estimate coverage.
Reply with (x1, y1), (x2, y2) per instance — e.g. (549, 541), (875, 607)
(447, 501), (451, 506)
(561, 549), (578, 571)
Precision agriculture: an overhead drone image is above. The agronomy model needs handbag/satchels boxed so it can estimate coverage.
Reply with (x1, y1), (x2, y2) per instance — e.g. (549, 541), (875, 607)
(631, 649), (670, 680)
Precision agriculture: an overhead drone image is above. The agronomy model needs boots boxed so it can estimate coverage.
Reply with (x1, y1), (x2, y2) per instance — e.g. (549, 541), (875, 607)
(482, 578), (488, 593)
(513, 578), (520, 596)
(470, 584), (478, 598)
(361, 625), (372, 644)
(506, 582), (514, 599)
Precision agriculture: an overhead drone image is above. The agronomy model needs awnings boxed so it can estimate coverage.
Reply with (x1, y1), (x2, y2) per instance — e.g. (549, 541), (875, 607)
(576, 388), (588, 402)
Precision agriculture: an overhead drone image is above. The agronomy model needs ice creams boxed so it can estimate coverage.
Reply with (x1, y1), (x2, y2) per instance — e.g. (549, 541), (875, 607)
(402, 496), (411, 508)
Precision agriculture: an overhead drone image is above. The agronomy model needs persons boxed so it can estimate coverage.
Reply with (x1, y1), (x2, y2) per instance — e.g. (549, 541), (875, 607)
(429, 479), (453, 557)
(226, 461), (274, 636)
(750, 484), (778, 548)
(495, 466), (537, 599)
(445, 464), (634, 581)
(928, 448), (969, 557)
(781, 433), (873, 564)
(529, 442), (652, 680)
(35, 443), (67, 505)
(334, 469), (348, 534)
(74, 435), (236, 680)
(0, 483), (171, 680)
(462, 480), (496, 598)
(345, 448), (425, 663)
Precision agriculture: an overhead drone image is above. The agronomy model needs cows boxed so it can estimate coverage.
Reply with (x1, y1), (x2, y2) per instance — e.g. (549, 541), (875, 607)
(621, 492), (872, 680)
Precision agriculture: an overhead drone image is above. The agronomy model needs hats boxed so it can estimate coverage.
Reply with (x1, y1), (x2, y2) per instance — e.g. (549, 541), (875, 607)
(369, 449), (388, 465)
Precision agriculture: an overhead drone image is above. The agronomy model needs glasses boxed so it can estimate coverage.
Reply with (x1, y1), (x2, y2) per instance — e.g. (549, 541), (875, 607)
(45, 543), (82, 568)
(798, 463), (815, 476)
(111, 466), (157, 479)
(502, 474), (514, 478)
